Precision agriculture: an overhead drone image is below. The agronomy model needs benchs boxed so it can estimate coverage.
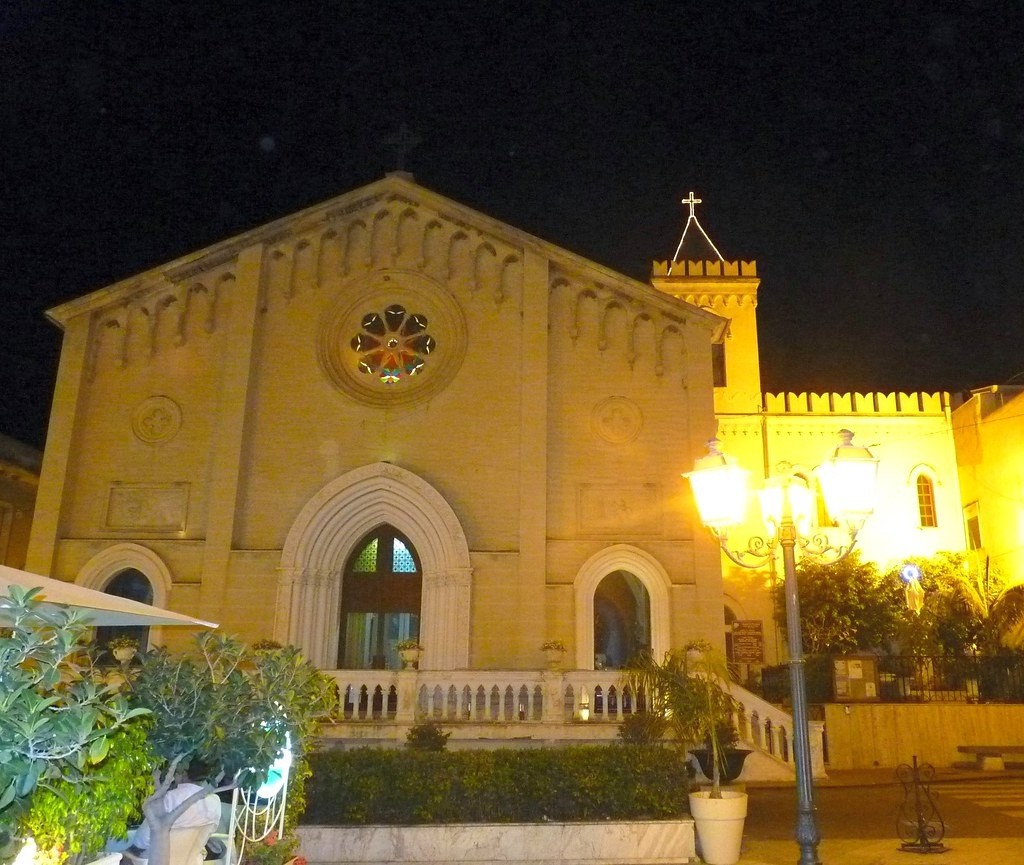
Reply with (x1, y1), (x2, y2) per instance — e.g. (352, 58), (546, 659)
(958, 745), (1024, 768)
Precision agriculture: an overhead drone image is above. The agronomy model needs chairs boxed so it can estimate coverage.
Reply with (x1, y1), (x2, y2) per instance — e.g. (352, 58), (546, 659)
(202, 802), (238, 865)
(121, 820), (218, 865)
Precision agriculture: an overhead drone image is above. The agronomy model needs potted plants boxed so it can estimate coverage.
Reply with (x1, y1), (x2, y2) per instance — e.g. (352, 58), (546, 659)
(398, 641), (424, 669)
(108, 635), (140, 670)
(620, 637), (748, 864)
(688, 717), (753, 784)
(541, 640), (566, 670)
(20, 697), (160, 865)
(253, 640), (283, 669)
